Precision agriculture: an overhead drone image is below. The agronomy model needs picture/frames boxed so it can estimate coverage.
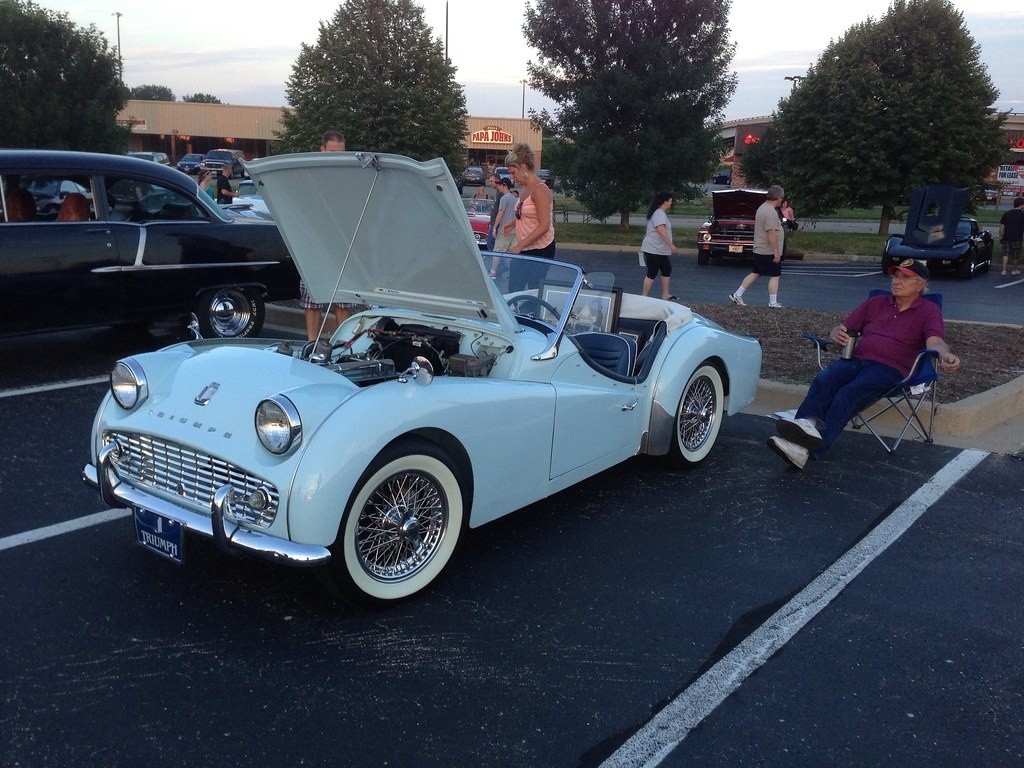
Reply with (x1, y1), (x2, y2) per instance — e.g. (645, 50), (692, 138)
(538, 278), (623, 339)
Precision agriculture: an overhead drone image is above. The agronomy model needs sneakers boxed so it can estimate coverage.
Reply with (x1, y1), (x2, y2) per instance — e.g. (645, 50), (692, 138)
(1000, 270), (1007, 277)
(775, 418), (823, 450)
(767, 436), (809, 470)
(1012, 270), (1021, 276)
(728, 293), (746, 306)
(768, 302), (784, 308)
(488, 270), (497, 279)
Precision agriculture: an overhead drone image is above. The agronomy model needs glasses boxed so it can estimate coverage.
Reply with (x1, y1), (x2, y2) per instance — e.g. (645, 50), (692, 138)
(515, 201), (523, 219)
(890, 275), (921, 283)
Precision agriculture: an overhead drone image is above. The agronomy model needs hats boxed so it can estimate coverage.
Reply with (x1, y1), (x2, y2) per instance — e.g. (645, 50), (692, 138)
(888, 259), (928, 281)
(495, 177), (511, 186)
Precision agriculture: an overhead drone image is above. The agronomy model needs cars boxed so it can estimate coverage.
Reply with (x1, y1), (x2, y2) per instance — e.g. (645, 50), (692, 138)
(177, 153), (207, 174)
(462, 167), (487, 185)
(495, 167), (516, 188)
(696, 188), (796, 267)
(536, 169), (554, 188)
(984, 189), (1003, 204)
(0, 149), (303, 347)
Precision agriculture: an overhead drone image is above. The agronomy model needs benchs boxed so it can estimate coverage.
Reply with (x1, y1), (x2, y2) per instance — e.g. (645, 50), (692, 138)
(110, 204), (203, 222)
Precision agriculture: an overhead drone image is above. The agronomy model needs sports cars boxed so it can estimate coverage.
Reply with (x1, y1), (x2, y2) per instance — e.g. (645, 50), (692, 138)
(882, 183), (993, 280)
(457, 198), (496, 248)
(84, 154), (763, 604)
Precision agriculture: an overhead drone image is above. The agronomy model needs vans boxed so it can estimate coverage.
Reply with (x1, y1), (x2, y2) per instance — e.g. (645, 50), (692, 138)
(126, 152), (171, 166)
(202, 149), (246, 179)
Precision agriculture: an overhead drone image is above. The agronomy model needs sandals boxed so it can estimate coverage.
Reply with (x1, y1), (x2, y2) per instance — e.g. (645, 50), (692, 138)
(663, 295), (680, 301)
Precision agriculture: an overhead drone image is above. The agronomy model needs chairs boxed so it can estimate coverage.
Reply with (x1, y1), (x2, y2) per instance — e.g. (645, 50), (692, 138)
(5, 187), (37, 223)
(56, 192), (89, 224)
(802, 289), (944, 456)
(564, 331), (632, 383)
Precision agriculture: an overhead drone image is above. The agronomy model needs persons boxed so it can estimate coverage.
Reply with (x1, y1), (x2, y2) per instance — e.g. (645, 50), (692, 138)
(505, 143), (555, 293)
(766, 258), (961, 473)
(487, 173), (505, 271)
(197, 170), (215, 200)
(299, 132), (361, 343)
(486, 178), (519, 278)
(641, 190), (681, 302)
(469, 186), (492, 211)
(999, 197), (1024, 276)
(729, 185), (785, 308)
(218, 165), (240, 204)
(780, 198), (795, 222)
(133, 180), (157, 203)
